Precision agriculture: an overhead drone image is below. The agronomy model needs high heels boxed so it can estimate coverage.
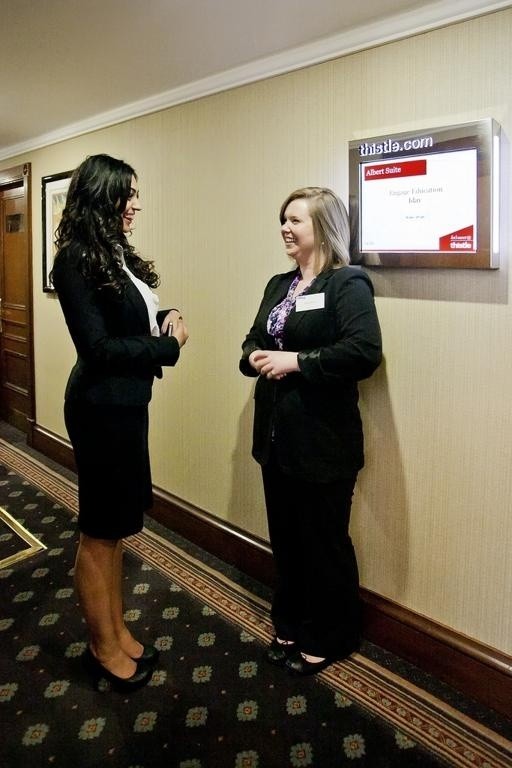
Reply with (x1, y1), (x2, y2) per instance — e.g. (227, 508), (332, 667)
(82, 641), (153, 688)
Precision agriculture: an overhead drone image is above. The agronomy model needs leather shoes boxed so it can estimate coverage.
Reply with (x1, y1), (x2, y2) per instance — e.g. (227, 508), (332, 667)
(133, 644), (158, 662)
(265, 637), (296, 665)
(284, 648), (339, 678)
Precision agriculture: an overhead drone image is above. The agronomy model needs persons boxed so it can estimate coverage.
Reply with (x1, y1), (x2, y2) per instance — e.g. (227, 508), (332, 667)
(52, 152), (190, 691)
(238, 185), (383, 674)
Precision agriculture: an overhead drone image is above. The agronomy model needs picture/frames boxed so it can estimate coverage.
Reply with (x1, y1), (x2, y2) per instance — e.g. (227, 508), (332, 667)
(347, 118), (502, 270)
(41, 167), (77, 294)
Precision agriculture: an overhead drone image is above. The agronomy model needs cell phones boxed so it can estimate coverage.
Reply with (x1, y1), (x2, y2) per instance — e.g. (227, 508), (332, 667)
(167, 322), (173, 337)
